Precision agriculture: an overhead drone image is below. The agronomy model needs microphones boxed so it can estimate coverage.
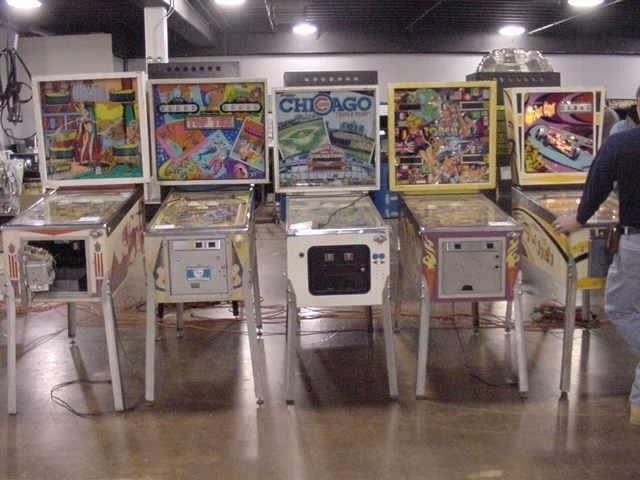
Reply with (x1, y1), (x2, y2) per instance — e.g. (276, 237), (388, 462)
(7, 31), (19, 53)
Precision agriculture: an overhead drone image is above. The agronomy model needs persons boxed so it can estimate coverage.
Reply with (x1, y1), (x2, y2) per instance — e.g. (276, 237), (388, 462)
(551, 88), (640, 425)
(601, 96), (640, 151)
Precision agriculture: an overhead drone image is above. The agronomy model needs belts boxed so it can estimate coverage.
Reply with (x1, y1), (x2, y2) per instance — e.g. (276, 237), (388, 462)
(618, 226), (638, 237)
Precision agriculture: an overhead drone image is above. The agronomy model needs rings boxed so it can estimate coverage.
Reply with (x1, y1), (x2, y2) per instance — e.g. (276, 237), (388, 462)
(555, 224), (560, 229)
